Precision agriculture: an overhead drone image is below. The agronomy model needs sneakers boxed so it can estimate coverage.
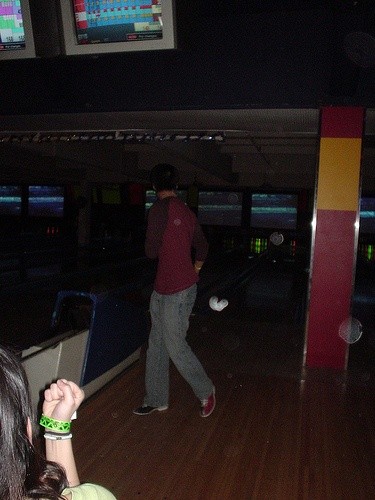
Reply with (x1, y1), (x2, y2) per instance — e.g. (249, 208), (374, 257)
(133, 405), (168, 415)
(199, 384), (216, 417)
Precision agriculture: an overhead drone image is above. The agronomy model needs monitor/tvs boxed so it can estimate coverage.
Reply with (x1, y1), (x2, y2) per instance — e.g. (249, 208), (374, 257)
(198, 187), (246, 228)
(144, 187), (189, 210)
(0, 184), (24, 217)
(359, 194), (375, 235)
(0, 0), (35, 60)
(60, 0), (178, 55)
(249, 190), (299, 230)
(27, 184), (69, 219)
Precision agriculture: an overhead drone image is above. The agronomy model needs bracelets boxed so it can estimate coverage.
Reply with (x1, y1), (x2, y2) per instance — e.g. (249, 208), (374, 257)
(39, 413), (71, 433)
(43, 431), (73, 440)
(194, 263), (202, 270)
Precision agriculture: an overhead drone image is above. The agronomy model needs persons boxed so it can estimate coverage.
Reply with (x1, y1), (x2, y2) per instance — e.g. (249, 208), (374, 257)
(0, 343), (118, 500)
(132, 162), (216, 419)
